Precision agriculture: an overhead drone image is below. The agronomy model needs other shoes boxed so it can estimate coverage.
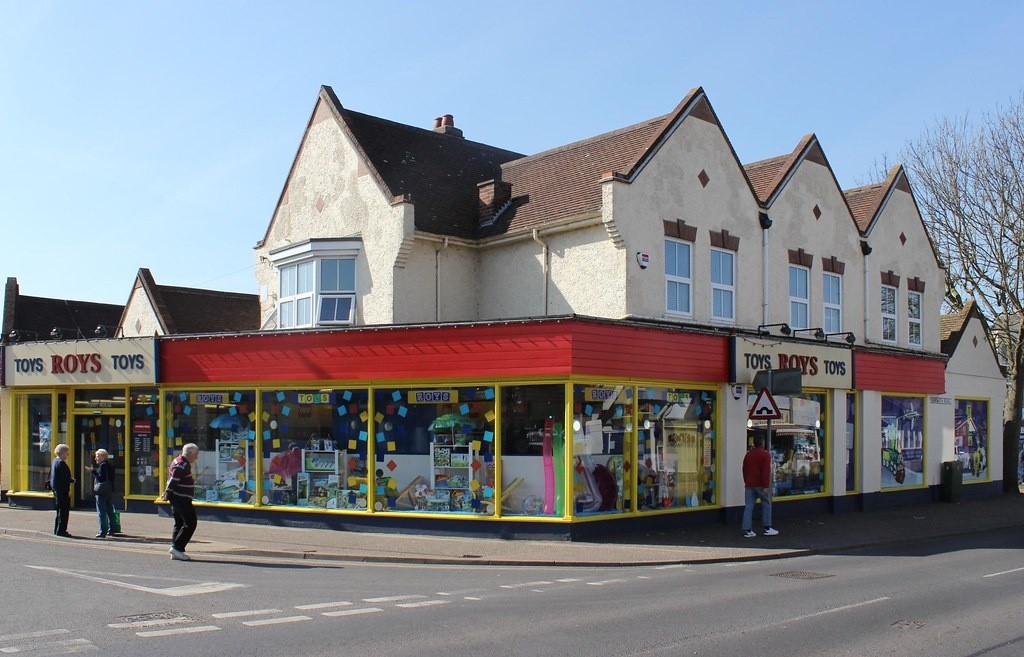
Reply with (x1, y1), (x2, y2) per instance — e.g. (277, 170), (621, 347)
(53, 531), (72, 538)
(94, 533), (106, 539)
(106, 534), (115, 537)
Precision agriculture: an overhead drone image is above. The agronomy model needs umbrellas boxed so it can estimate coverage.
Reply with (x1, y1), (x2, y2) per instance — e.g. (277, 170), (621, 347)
(210, 413), (252, 428)
(428, 413), (475, 448)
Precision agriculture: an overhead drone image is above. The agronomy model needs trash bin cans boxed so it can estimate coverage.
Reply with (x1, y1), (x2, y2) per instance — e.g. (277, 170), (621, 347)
(944, 461), (963, 504)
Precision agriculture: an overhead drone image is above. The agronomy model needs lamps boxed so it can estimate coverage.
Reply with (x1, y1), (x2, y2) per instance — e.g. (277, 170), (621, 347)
(794, 327), (824, 340)
(758, 323), (791, 336)
(826, 332), (855, 343)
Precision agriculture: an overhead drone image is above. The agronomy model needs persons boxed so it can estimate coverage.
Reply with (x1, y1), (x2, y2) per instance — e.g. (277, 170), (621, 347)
(85, 448), (120, 537)
(50, 444), (76, 538)
(161, 443), (199, 560)
(742, 434), (779, 537)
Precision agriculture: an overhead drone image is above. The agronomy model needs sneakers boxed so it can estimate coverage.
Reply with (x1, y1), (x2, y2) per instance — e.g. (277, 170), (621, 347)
(764, 528), (779, 535)
(744, 530), (757, 537)
(169, 547), (191, 560)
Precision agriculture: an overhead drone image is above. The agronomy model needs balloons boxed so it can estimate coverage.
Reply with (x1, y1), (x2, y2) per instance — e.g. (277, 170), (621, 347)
(541, 417), (564, 516)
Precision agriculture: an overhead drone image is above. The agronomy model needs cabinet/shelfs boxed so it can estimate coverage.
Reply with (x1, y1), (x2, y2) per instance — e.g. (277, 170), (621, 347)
(216, 439), (249, 482)
(301, 448), (347, 496)
(430, 442), (479, 494)
